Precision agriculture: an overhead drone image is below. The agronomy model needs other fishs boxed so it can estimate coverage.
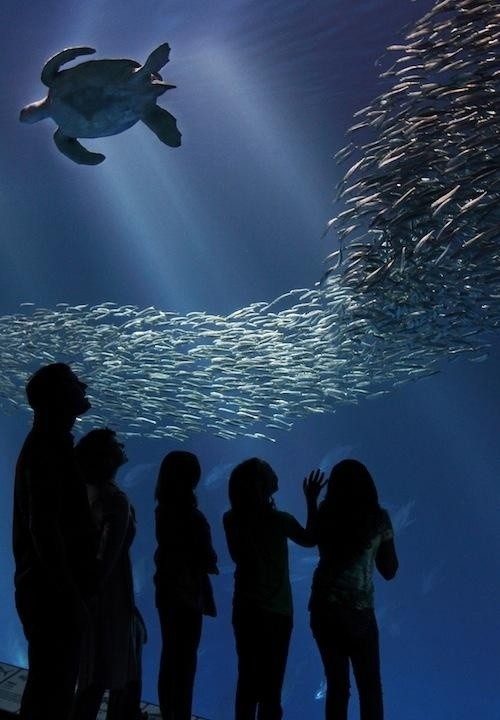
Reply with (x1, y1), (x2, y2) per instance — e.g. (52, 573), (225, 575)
(318, 1), (499, 402)
(1, 291), (339, 443)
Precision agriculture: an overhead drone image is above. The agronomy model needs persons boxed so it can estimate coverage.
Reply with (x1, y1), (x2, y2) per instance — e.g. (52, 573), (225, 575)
(8, 360), (100, 719)
(148, 448), (222, 720)
(309, 459), (401, 720)
(221, 454), (327, 719)
(72, 426), (151, 719)
